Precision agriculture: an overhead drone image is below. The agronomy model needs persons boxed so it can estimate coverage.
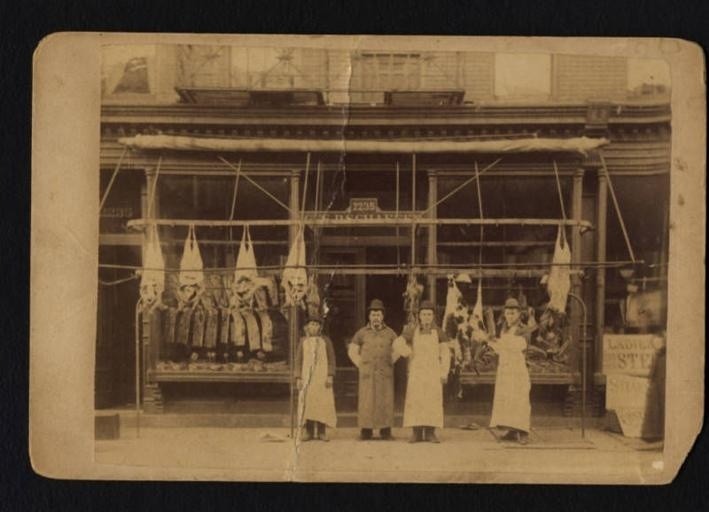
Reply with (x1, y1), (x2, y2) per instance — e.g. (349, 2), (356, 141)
(292, 298), (535, 445)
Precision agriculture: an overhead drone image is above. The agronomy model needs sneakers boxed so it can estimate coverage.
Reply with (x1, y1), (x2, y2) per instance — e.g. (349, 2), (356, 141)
(301, 431), (330, 442)
(357, 431), (395, 440)
(500, 430), (529, 443)
(409, 430), (439, 443)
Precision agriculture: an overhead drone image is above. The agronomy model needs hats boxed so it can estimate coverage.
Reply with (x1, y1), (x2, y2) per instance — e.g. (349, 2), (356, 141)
(417, 301), (436, 311)
(504, 298), (522, 311)
(366, 299), (386, 313)
(305, 314), (325, 324)
